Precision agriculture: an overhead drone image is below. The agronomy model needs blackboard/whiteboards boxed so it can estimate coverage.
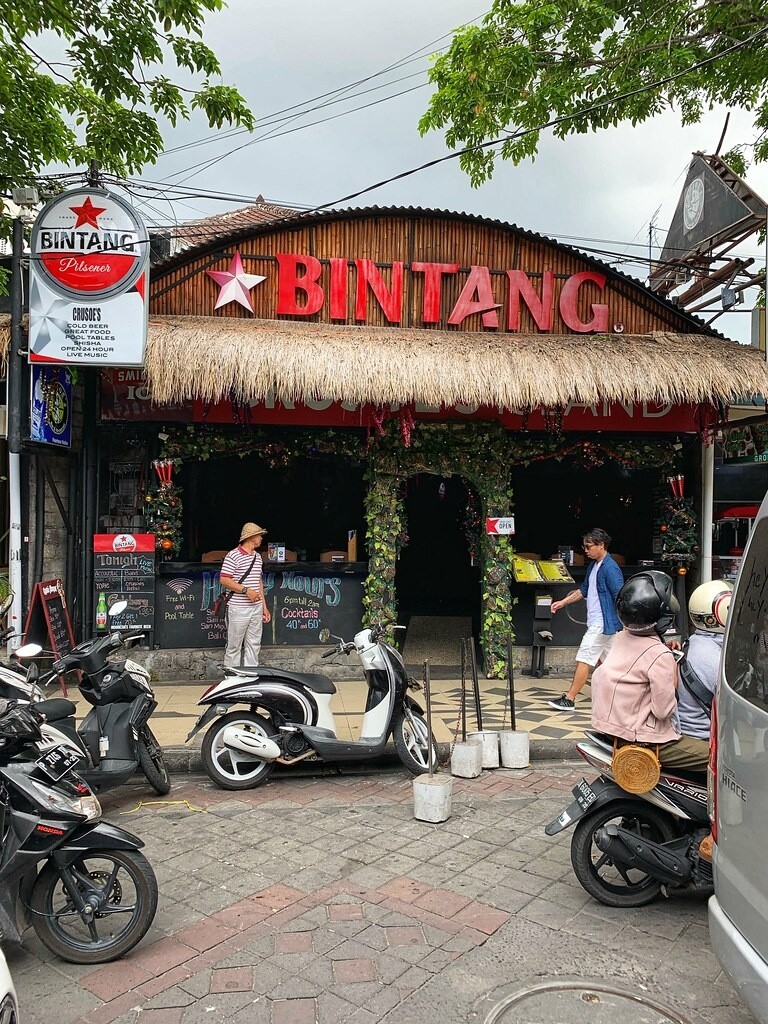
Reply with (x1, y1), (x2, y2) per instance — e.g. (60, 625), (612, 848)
(17, 579), (75, 664)
(93, 551), (155, 632)
(149, 572), (368, 650)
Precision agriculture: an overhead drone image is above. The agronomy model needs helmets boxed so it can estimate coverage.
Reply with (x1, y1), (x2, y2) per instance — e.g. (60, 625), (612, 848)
(688, 579), (736, 633)
(616, 570), (679, 636)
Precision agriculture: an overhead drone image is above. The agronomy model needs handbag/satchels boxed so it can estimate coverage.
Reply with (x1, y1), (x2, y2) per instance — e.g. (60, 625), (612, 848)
(213, 592), (228, 620)
(612, 743), (661, 794)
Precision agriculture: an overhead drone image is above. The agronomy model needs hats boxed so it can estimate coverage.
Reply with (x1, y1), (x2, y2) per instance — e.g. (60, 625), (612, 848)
(239, 523), (268, 542)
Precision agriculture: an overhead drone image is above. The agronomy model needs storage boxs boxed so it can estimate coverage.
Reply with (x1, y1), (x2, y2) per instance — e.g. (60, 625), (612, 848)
(638, 559), (655, 566)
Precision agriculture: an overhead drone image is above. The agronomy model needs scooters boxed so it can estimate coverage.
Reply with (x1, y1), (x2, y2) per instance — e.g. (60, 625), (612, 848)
(184, 585), (441, 790)
(17, 600), (170, 798)
(0, 594), (159, 966)
(544, 731), (715, 909)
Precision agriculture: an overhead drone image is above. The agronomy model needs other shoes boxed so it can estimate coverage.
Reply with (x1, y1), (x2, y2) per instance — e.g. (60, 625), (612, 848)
(699, 833), (714, 863)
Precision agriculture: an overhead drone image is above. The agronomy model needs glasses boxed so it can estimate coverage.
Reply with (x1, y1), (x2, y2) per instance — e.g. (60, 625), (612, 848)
(581, 543), (599, 550)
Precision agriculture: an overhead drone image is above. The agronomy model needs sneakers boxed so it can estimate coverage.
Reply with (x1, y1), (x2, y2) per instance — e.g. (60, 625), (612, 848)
(548, 692), (576, 710)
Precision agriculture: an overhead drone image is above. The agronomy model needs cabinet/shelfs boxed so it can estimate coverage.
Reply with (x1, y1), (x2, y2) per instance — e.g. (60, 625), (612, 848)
(105, 427), (163, 533)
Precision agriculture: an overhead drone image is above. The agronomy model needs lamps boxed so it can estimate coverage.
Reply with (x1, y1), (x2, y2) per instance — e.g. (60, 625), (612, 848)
(674, 270), (693, 284)
(719, 285), (736, 310)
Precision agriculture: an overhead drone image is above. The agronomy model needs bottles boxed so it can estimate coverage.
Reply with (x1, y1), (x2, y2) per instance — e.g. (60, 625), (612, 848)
(96, 592), (107, 630)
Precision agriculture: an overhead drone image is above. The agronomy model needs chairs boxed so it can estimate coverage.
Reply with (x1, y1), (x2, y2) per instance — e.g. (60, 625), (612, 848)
(320, 551), (349, 561)
(517, 550), (542, 559)
(610, 553), (626, 564)
(262, 549), (298, 563)
(550, 553), (586, 565)
(199, 551), (228, 562)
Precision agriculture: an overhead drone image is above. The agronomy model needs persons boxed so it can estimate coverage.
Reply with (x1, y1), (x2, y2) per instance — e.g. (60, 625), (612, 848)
(220, 523), (271, 670)
(669, 578), (735, 742)
(592, 569), (709, 772)
(548, 528), (622, 710)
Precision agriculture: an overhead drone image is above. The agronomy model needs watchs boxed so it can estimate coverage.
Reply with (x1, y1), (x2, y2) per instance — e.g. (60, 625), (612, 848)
(242, 587), (247, 593)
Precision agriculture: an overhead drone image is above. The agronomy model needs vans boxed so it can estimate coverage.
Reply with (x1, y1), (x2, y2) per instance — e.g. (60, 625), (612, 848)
(706, 489), (767, 1024)
